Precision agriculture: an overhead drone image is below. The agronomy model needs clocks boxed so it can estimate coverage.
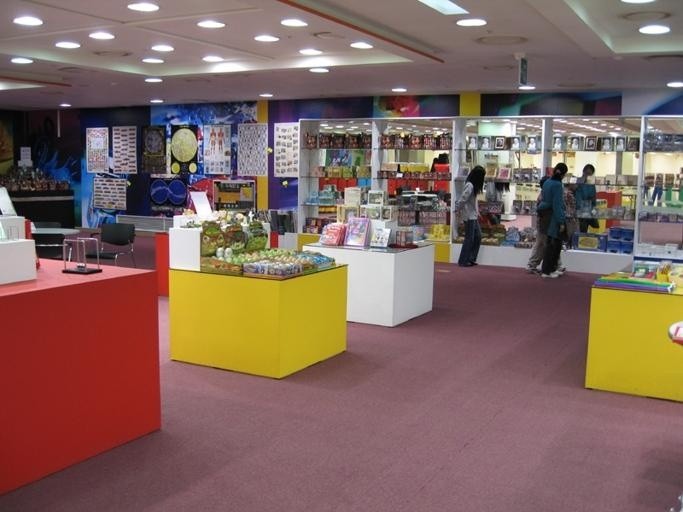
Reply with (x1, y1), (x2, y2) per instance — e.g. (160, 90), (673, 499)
(142, 124), (166, 175)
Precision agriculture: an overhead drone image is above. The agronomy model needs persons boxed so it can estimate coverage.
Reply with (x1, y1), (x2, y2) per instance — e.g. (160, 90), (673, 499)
(588, 140), (593, 147)
(511, 138), (518, 148)
(467, 138), (477, 149)
(575, 164), (601, 232)
(539, 163), (569, 278)
(452, 165), (485, 267)
(497, 140), (502, 147)
(554, 139), (561, 149)
(528, 138), (535, 149)
(602, 140), (610, 150)
(525, 176), (566, 274)
(571, 139), (578, 149)
(485, 175), (510, 226)
(616, 139), (623, 150)
(479, 138), (489, 149)
(564, 185), (579, 249)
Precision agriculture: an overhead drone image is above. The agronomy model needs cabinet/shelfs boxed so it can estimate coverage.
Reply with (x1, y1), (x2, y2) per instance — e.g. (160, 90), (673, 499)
(372, 118), (454, 246)
(544, 116), (643, 257)
(298, 118), (375, 233)
(636, 117), (682, 260)
(453, 117), (544, 250)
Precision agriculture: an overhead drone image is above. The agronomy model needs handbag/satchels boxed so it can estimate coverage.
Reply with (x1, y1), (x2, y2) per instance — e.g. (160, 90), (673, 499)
(562, 222), (574, 242)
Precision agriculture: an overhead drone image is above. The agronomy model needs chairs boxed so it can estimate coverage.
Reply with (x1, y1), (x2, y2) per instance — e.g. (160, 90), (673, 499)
(31, 233), (73, 262)
(85, 223), (136, 267)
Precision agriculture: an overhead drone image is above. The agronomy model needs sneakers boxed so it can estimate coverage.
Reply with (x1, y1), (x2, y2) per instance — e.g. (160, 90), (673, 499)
(459, 262), (478, 266)
(526, 265), (565, 278)
